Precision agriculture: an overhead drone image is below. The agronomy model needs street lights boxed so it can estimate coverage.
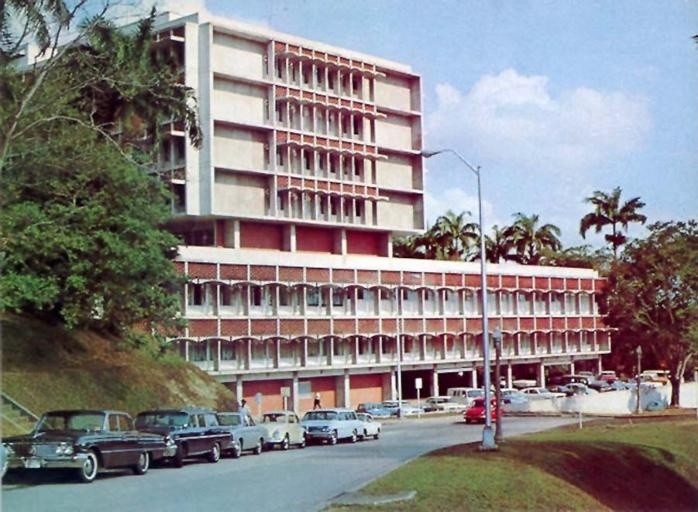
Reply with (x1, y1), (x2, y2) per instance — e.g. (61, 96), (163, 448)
(421, 146), (498, 452)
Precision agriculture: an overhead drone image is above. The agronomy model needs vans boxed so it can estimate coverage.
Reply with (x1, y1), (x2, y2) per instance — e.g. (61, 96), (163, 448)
(301, 409), (364, 445)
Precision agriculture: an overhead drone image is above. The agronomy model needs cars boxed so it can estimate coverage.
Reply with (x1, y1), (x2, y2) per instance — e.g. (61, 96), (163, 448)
(358, 401), (425, 418)
(2, 410), (179, 483)
(356, 413), (381, 440)
(262, 412), (306, 450)
(215, 412), (269, 458)
(135, 406), (235, 468)
(426, 368), (668, 423)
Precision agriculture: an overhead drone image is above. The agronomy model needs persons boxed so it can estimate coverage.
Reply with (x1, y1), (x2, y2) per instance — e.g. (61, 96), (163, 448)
(240, 398), (251, 414)
(312, 391), (322, 409)
